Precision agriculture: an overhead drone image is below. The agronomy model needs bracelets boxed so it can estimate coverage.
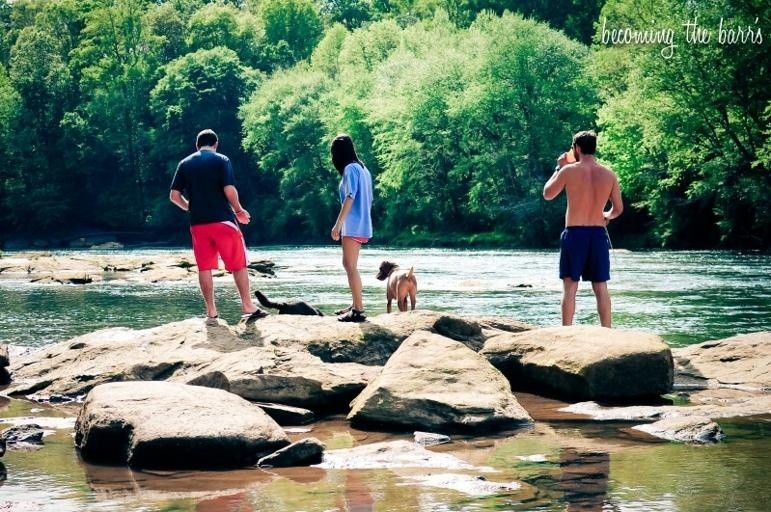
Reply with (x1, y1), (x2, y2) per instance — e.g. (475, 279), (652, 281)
(235, 210), (243, 214)
(555, 165), (562, 170)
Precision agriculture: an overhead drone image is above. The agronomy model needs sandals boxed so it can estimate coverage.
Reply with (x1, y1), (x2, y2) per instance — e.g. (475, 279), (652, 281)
(335, 304), (368, 322)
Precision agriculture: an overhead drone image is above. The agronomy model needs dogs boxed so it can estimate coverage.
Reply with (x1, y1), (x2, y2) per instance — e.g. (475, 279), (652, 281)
(376, 260), (419, 314)
(253, 290), (325, 315)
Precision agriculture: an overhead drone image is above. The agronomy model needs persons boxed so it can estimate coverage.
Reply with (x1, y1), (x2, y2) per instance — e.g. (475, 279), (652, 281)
(169, 129), (270, 319)
(327, 133), (373, 322)
(541, 130), (625, 329)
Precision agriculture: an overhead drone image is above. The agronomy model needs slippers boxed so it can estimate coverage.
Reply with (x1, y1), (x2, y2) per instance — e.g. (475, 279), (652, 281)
(239, 307), (271, 318)
